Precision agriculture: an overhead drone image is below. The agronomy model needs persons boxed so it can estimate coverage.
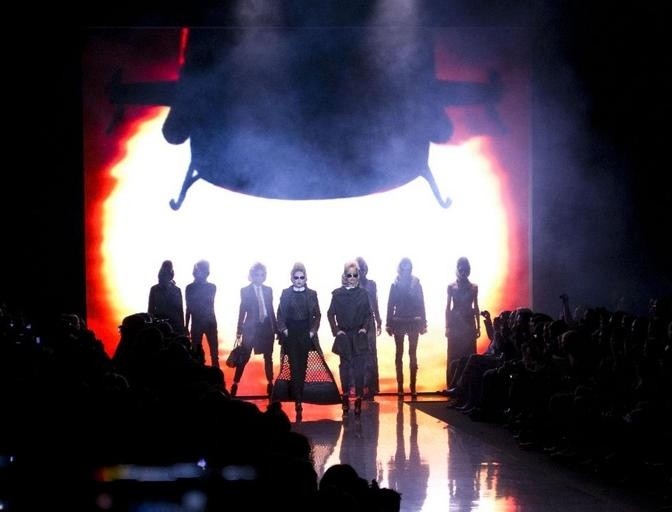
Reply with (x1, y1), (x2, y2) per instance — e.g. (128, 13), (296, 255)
(385, 258), (428, 397)
(446, 294), (672, 494)
(1, 314), (401, 511)
(280, 263), (320, 411)
(149, 260), (183, 324)
(354, 257), (383, 402)
(186, 263), (221, 366)
(328, 263), (368, 415)
(445, 257), (482, 382)
(230, 263), (276, 395)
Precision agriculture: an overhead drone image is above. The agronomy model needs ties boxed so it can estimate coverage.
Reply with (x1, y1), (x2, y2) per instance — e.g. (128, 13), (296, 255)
(257, 287), (265, 324)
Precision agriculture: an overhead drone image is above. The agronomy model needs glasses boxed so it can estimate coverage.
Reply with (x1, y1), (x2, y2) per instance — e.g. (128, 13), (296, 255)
(293, 276), (305, 280)
(346, 273), (358, 278)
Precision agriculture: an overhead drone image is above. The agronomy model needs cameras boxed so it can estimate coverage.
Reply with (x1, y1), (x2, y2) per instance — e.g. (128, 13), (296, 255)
(481, 312), (487, 318)
(559, 294), (568, 303)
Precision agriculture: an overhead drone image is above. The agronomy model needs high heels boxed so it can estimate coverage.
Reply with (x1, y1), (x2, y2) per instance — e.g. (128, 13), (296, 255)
(342, 399), (349, 412)
(354, 398), (361, 416)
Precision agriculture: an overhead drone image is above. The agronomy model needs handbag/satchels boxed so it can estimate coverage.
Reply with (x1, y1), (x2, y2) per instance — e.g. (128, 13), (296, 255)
(226, 338), (242, 368)
